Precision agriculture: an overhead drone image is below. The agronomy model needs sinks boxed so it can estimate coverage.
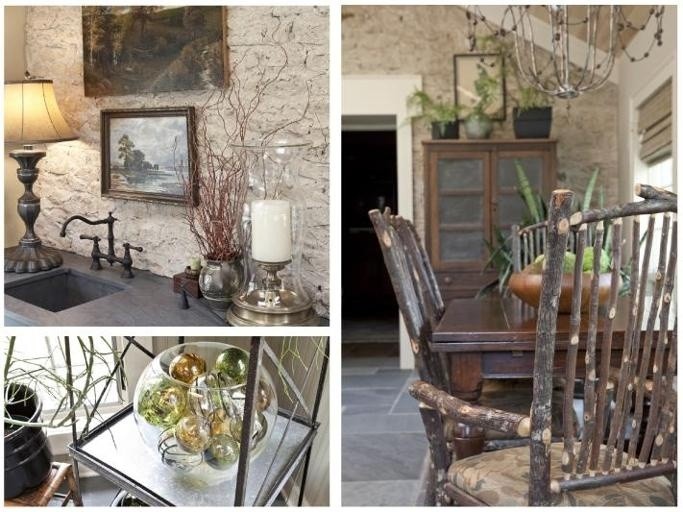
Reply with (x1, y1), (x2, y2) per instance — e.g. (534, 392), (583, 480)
(4, 267), (132, 313)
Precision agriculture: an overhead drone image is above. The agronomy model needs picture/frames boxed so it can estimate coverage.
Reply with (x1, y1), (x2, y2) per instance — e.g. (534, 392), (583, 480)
(452, 52), (506, 124)
(99, 105), (199, 207)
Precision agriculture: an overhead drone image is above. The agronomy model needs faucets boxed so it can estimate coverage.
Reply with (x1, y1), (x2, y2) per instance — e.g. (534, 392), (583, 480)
(59, 211), (120, 267)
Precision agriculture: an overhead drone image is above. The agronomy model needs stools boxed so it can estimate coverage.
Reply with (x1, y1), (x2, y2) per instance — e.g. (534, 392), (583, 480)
(4, 461), (86, 507)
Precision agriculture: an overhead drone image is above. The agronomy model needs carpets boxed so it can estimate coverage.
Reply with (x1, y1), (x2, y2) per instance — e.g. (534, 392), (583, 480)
(342, 318), (401, 344)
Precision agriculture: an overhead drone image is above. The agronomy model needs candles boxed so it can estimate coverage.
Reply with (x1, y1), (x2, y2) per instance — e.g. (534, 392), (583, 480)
(250, 198), (294, 263)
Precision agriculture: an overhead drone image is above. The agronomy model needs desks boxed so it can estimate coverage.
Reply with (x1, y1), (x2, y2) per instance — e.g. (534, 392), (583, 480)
(430, 295), (677, 461)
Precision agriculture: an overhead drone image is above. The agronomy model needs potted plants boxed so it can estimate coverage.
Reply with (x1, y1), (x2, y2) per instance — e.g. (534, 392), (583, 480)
(511, 81), (553, 139)
(4, 335), (118, 500)
(168, 44), (300, 303)
(508, 246), (625, 314)
(408, 86), (464, 140)
(463, 63), (511, 140)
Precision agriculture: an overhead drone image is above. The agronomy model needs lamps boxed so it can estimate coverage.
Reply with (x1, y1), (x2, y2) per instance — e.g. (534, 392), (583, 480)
(4, 70), (83, 275)
(458, 5), (669, 102)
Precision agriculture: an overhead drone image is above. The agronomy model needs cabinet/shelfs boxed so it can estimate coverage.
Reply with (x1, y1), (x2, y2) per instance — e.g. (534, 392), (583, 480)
(420, 138), (560, 300)
(63, 335), (329, 508)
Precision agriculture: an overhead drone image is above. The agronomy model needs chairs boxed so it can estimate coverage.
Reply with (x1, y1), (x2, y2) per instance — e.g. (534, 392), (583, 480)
(368, 207), (584, 506)
(406, 181), (677, 506)
(396, 217), (446, 312)
(510, 217), (577, 299)
